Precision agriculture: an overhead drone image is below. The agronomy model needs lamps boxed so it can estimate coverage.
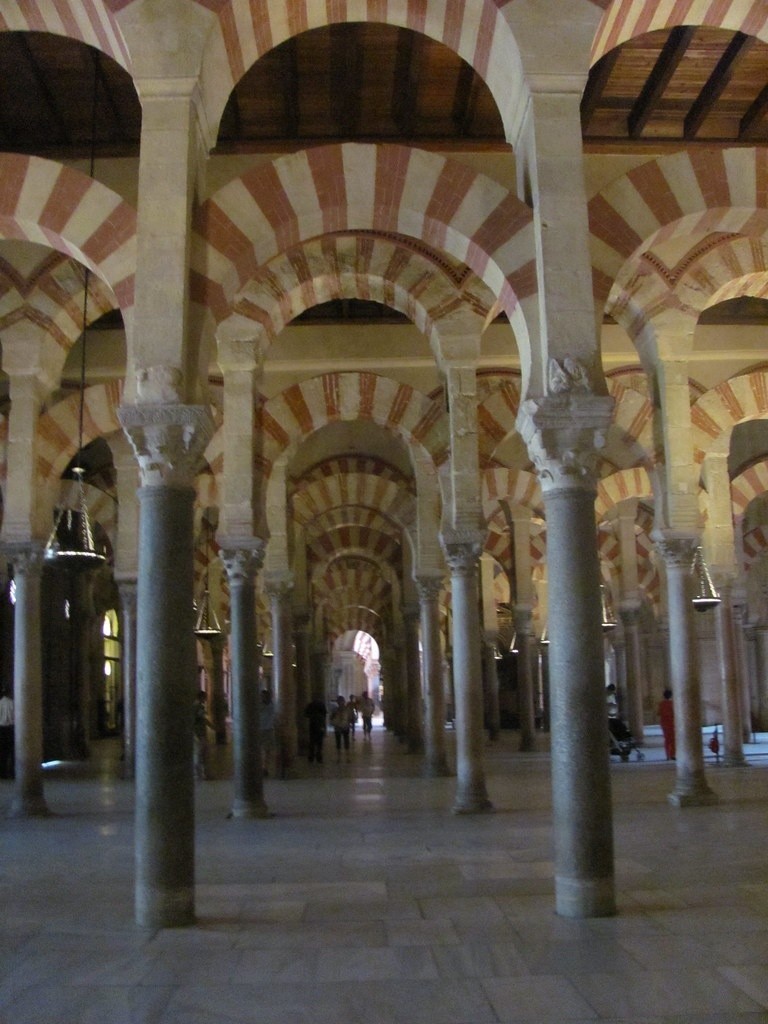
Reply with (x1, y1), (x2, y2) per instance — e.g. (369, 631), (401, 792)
(44, 54), (108, 574)
(508, 633), (519, 654)
(540, 623), (550, 646)
(193, 508), (220, 639)
(601, 588), (616, 633)
(690, 548), (722, 611)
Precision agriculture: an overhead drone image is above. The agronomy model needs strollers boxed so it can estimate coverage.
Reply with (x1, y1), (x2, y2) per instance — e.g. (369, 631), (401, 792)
(606, 717), (645, 764)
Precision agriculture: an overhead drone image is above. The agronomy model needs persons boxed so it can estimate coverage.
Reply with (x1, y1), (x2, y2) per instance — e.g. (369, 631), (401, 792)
(217, 693), (230, 745)
(657, 690), (676, 760)
(605, 683), (619, 719)
(192, 690), (218, 779)
(358, 692), (376, 740)
(259, 690), (275, 778)
(330, 696), (352, 763)
(0, 690), (15, 763)
(113, 694), (124, 761)
(347, 694), (359, 742)
(303, 692), (328, 763)
